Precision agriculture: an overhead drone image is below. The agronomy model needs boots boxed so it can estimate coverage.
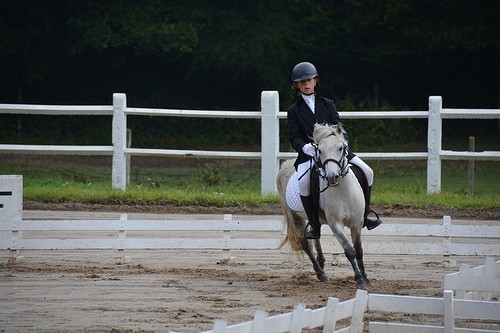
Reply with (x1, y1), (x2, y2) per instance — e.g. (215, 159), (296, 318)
(300, 194), (321, 239)
(362, 186), (382, 230)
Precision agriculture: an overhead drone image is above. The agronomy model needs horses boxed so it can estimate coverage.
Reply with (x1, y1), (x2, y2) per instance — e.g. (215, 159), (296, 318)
(274, 121), (374, 290)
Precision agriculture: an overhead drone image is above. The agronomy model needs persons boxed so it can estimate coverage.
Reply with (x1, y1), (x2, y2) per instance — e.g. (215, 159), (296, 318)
(287, 62), (382, 239)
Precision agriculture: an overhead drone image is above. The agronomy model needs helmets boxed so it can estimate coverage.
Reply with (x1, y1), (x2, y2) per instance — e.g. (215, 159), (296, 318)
(292, 61), (318, 82)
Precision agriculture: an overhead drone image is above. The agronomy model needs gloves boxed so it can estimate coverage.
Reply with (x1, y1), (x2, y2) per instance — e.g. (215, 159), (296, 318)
(302, 143), (318, 157)
